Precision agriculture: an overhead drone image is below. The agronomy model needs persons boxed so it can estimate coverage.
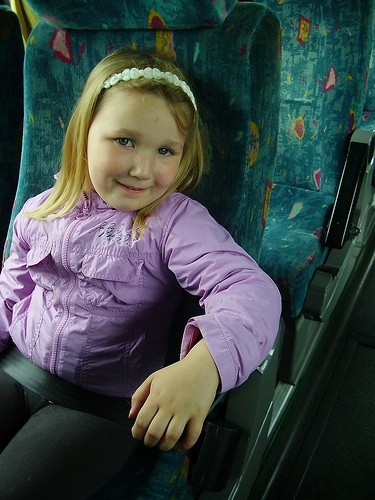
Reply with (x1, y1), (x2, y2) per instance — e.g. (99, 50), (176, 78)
(0, 45), (283, 500)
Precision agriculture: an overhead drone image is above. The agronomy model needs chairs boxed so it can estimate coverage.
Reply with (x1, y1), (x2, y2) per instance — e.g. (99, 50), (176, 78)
(0, 5), (25, 276)
(259, 0), (374, 388)
(0, 1), (287, 500)
(358, 49), (374, 210)
